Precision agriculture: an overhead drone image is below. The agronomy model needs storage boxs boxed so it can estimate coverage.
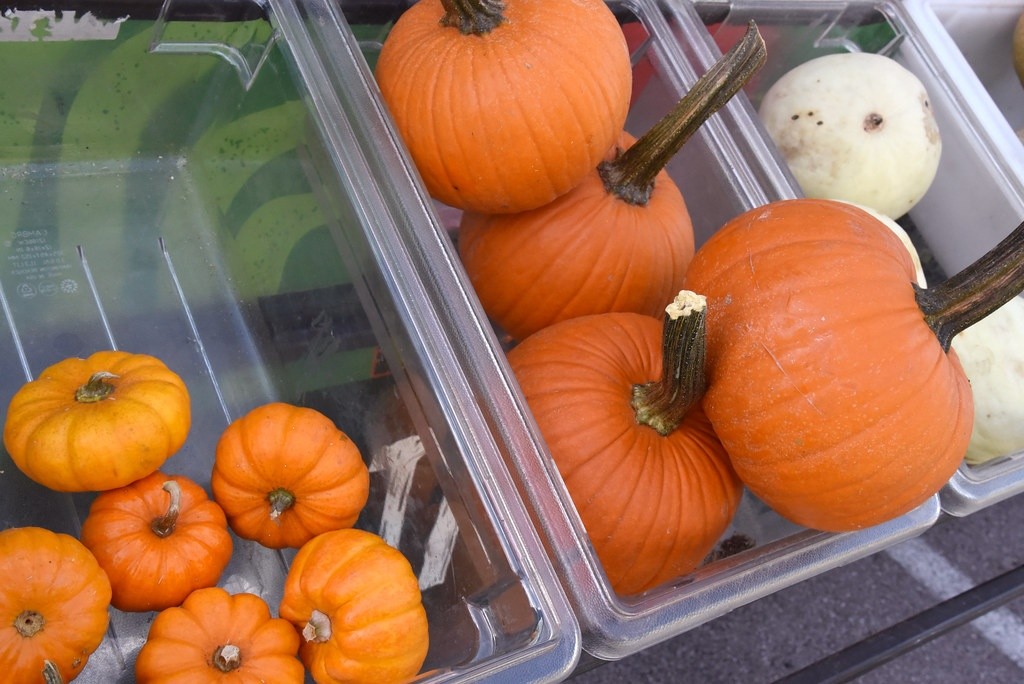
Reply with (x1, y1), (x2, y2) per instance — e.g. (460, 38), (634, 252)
(0, 0), (1024, 684)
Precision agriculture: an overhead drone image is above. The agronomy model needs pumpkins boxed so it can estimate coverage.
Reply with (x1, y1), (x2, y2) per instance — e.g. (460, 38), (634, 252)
(0, 350), (430, 684)
(375, 0), (1024, 601)
